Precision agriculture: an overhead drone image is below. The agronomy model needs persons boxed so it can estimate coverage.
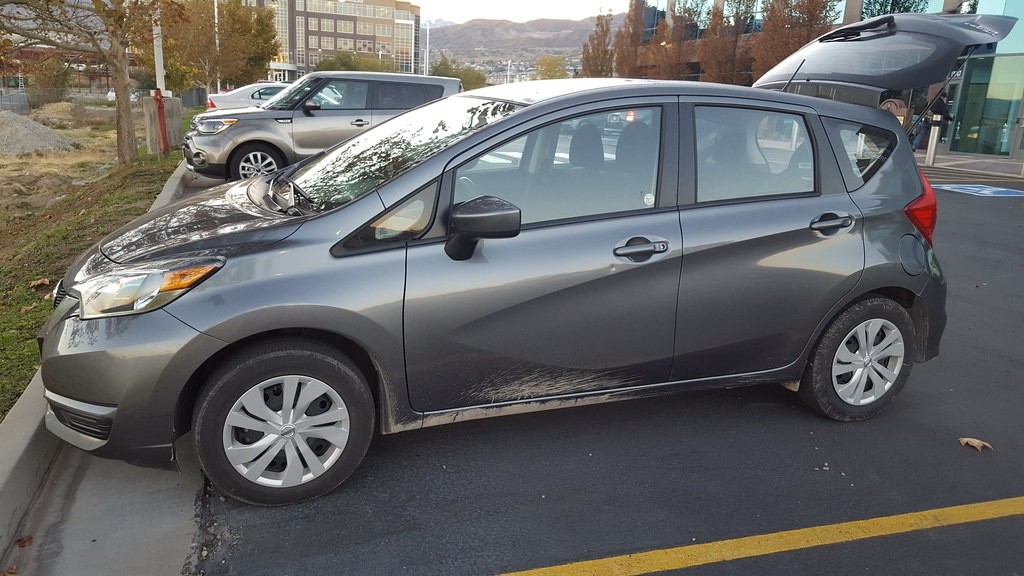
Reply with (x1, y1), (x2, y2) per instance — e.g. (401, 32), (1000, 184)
(912, 92), (954, 153)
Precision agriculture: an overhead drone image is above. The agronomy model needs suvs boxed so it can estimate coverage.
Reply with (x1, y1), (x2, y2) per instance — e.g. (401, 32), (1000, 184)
(179, 70), (467, 182)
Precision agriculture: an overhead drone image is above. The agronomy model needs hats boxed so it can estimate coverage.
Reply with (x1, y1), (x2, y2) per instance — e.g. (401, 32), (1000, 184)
(940, 92), (948, 98)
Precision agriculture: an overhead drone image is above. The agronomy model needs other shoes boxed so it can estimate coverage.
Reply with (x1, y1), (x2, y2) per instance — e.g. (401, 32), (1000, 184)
(912, 149), (916, 152)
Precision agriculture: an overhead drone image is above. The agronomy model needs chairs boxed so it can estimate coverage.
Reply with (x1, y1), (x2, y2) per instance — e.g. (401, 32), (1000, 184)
(755, 142), (813, 194)
(595, 120), (654, 212)
(699, 126), (751, 198)
(524, 123), (608, 218)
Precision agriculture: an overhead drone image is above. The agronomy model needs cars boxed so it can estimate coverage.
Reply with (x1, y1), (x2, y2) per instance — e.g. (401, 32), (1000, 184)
(31, 10), (1021, 510)
(321, 86), (341, 102)
(203, 82), (341, 111)
